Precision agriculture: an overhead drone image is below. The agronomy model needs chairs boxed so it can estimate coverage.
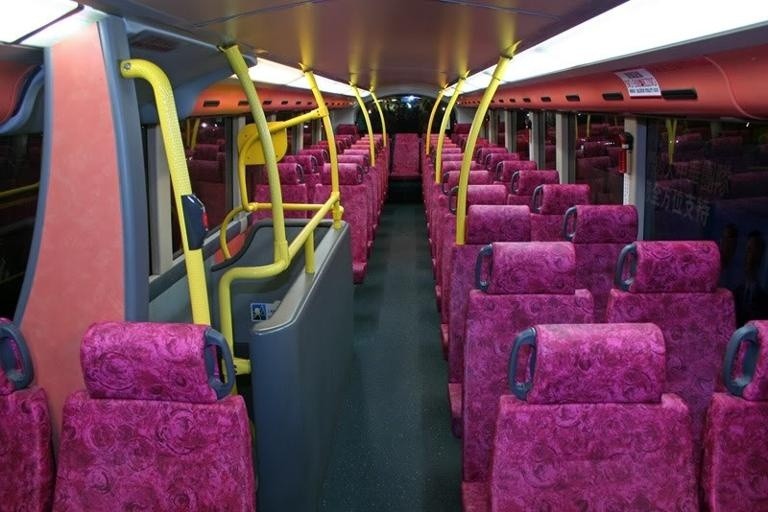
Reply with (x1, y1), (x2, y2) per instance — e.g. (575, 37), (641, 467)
(48, 318), (256, 511)
(0, 312), (56, 511)
(488, 321), (698, 512)
(603, 238), (734, 479)
(448, 204), (530, 425)
(460, 241), (597, 512)
(563, 203), (638, 319)
(698, 318), (768, 511)
